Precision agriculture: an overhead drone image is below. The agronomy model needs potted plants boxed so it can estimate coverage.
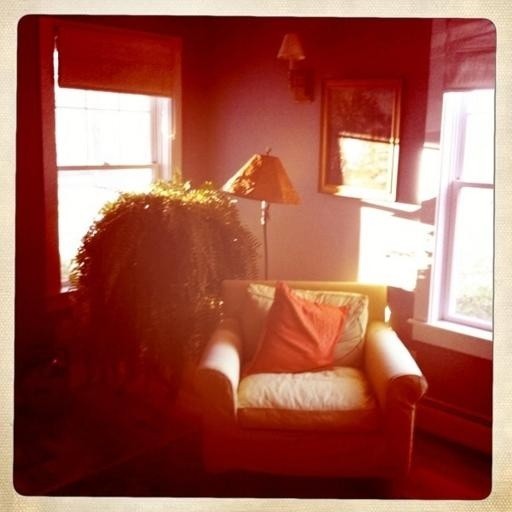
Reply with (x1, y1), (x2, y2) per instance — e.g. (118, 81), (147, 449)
(71, 174), (261, 368)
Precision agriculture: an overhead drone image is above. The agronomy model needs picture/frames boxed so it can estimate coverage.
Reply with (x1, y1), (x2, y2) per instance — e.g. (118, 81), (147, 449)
(318, 77), (407, 203)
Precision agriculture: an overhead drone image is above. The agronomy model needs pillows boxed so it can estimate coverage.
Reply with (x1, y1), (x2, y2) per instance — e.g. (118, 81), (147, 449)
(241, 279), (348, 377)
(245, 283), (369, 368)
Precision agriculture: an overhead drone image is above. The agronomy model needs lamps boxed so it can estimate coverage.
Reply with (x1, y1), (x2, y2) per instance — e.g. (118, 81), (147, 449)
(277, 31), (312, 103)
(221, 148), (302, 279)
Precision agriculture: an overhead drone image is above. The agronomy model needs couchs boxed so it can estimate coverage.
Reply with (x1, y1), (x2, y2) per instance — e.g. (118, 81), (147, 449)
(195, 280), (429, 482)
